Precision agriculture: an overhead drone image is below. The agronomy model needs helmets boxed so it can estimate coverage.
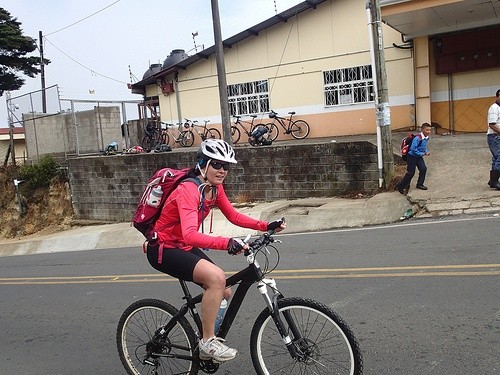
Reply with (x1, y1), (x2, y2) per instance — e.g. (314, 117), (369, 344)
(197, 139), (238, 164)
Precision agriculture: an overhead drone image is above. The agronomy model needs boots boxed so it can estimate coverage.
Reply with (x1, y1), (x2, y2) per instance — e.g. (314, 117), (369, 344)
(488, 170), (500, 190)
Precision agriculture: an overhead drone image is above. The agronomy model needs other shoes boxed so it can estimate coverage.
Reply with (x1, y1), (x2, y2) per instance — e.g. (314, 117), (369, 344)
(396, 183), (403, 194)
(417, 184), (428, 190)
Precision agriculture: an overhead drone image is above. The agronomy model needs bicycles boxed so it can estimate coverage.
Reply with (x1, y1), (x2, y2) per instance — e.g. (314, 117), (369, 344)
(231, 115), (270, 144)
(264, 109), (310, 140)
(142, 120), (195, 152)
(179, 120), (222, 147)
(116, 217), (364, 375)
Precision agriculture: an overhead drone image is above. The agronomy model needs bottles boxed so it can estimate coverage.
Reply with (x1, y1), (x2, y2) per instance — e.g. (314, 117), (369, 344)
(214, 298), (228, 334)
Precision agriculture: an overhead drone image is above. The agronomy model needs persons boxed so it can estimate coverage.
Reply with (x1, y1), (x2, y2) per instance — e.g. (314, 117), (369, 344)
(487, 89), (500, 189)
(147, 139), (286, 360)
(397, 123), (431, 194)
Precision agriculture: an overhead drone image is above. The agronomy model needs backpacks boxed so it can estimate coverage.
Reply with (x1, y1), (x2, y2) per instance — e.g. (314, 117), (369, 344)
(132, 168), (217, 247)
(401, 134), (422, 161)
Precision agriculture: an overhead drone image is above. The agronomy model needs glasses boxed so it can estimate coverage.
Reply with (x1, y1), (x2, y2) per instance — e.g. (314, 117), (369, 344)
(207, 161), (230, 171)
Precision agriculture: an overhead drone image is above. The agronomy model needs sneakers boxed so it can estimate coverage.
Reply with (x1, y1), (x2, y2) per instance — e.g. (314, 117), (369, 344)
(199, 337), (238, 361)
